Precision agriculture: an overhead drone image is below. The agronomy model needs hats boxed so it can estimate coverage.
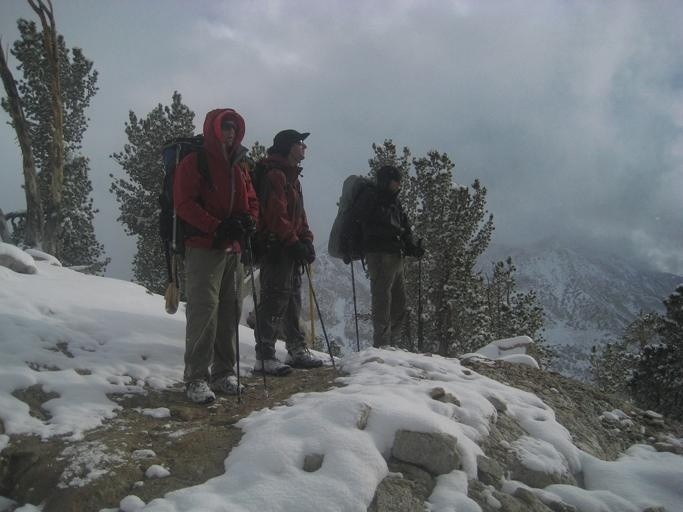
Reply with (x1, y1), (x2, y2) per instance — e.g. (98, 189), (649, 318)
(272, 129), (312, 150)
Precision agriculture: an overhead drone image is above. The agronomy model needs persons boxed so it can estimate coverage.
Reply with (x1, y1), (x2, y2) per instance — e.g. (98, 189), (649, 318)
(254, 129), (326, 378)
(348, 165), (427, 352)
(173, 107), (262, 405)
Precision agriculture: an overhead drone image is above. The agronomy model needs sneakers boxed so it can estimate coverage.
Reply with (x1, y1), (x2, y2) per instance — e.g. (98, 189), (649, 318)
(287, 346), (325, 371)
(252, 357), (296, 377)
(183, 377), (216, 404)
(213, 376), (246, 395)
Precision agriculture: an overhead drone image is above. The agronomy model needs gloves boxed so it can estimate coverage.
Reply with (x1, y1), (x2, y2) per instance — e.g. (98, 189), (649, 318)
(291, 238), (317, 265)
(219, 212), (258, 243)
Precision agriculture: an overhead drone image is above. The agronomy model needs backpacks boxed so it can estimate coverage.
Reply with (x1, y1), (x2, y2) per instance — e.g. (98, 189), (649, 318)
(157, 133), (203, 248)
(326, 174), (370, 264)
